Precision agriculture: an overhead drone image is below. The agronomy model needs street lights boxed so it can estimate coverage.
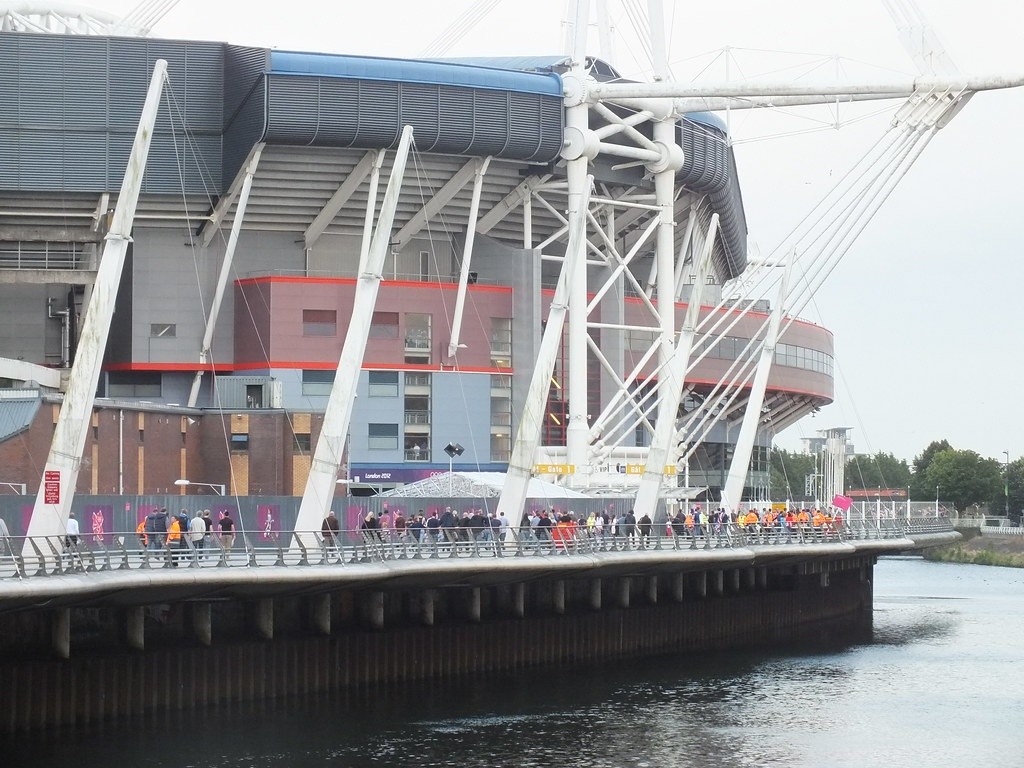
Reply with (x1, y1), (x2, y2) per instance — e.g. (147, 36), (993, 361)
(174, 480), (226, 496)
(336, 479), (383, 496)
(1002, 450), (1009, 518)
(443, 442), (465, 496)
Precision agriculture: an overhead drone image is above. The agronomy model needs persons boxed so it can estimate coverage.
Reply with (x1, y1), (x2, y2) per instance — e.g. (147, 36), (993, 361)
(666, 507), (843, 540)
(520, 508), (655, 550)
(64, 512), (80, 560)
(0, 517), (13, 564)
(321, 511), (339, 557)
(136, 507), (215, 566)
(361, 507), (510, 555)
(217, 510), (235, 561)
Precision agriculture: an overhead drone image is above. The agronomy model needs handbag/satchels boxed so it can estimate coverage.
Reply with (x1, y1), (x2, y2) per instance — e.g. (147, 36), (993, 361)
(76, 537), (82, 546)
